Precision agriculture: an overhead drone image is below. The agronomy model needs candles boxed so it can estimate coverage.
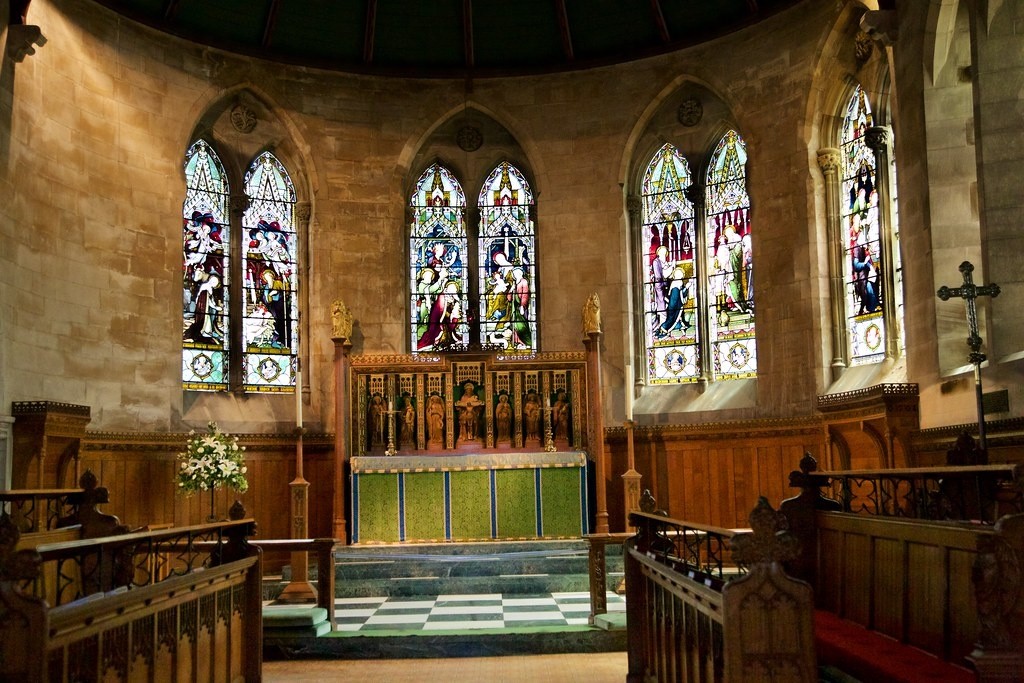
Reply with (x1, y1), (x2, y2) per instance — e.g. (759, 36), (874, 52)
(624, 364), (632, 421)
(295, 371), (303, 428)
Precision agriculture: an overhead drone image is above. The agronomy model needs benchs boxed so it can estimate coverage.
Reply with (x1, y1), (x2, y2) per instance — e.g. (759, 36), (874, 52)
(132, 534), (343, 630)
(578, 525), (754, 630)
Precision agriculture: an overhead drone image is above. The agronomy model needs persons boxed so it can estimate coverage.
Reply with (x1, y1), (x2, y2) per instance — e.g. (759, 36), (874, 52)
(496, 394), (512, 443)
(426, 394), (445, 442)
(524, 392), (542, 440)
(552, 392), (571, 442)
(332, 300), (347, 334)
(369, 396), (386, 446)
(455, 383), (483, 442)
(587, 293), (601, 332)
(398, 397), (416, 445)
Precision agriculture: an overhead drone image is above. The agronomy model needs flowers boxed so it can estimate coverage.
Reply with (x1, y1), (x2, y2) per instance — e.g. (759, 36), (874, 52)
(169, 417), (251, 500)
